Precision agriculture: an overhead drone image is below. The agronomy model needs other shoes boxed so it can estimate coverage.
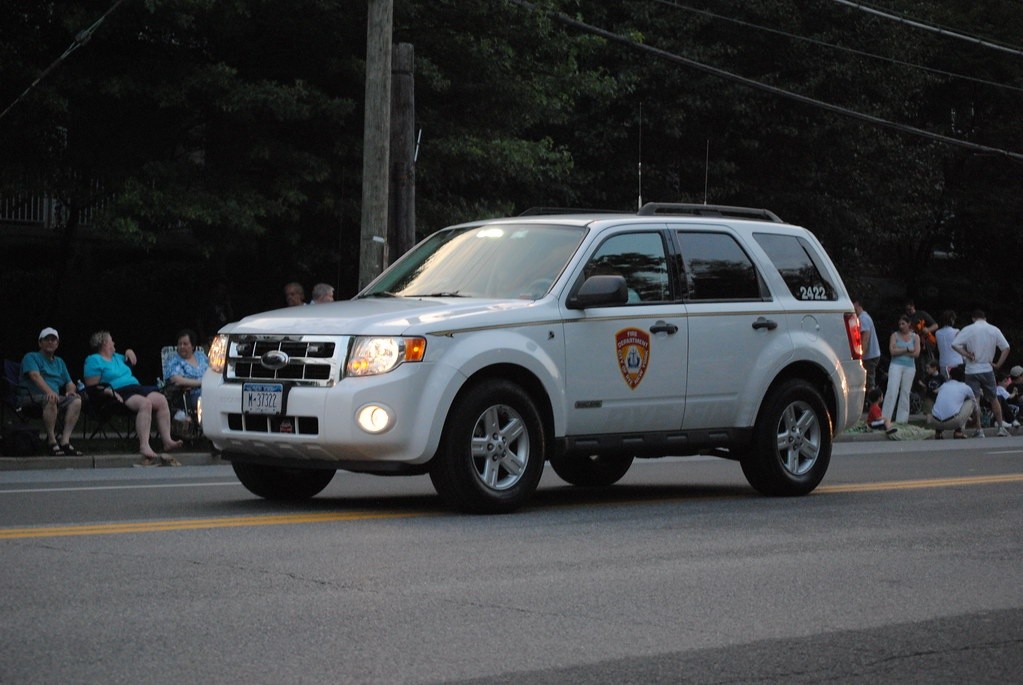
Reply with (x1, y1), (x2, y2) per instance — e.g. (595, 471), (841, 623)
(994, 420), (1012, 428)
(1013, 419), (1021, 427)
(953, 431), (968, 439)
(935, 432), (944, 439)
(996, 427), (1007, 437)
(972, 429), (985, 438)
(886, 427), (898, 434)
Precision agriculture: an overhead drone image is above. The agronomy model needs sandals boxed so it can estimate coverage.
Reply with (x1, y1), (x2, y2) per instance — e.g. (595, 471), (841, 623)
(45, 438), (64, 456)
(58, 442), (83, 456)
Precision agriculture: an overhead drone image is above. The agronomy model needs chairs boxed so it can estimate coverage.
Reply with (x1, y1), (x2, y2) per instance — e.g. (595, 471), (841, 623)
(0, 346), (204, 446)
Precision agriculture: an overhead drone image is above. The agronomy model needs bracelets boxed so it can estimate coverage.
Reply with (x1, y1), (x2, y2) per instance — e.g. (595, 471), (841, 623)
(906, 347), (909, 353)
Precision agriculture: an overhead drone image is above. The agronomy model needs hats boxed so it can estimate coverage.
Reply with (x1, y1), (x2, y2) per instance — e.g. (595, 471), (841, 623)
(38, 327), (59, 340)
(1010, 366), (1023, 376)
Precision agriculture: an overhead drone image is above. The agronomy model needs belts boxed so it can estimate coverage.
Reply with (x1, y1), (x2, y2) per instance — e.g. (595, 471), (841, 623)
(933, 415), (954, 423)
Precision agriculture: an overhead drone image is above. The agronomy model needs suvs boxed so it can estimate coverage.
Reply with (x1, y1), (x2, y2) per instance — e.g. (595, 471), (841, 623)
(198, 201), (870, 518)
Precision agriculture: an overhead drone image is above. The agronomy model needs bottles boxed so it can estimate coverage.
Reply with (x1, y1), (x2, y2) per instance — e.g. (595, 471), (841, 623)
(77, 379), (85, 391)
(156, 377), (165, 389)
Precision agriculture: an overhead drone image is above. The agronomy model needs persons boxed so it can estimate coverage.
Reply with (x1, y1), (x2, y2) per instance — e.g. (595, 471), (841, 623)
(282, 282), (334, 305)
(163, 328), (208, 407)
(16, 327), (82, 456)
(852, 299), (1022, 439)
(82, 330), (183, 458)
